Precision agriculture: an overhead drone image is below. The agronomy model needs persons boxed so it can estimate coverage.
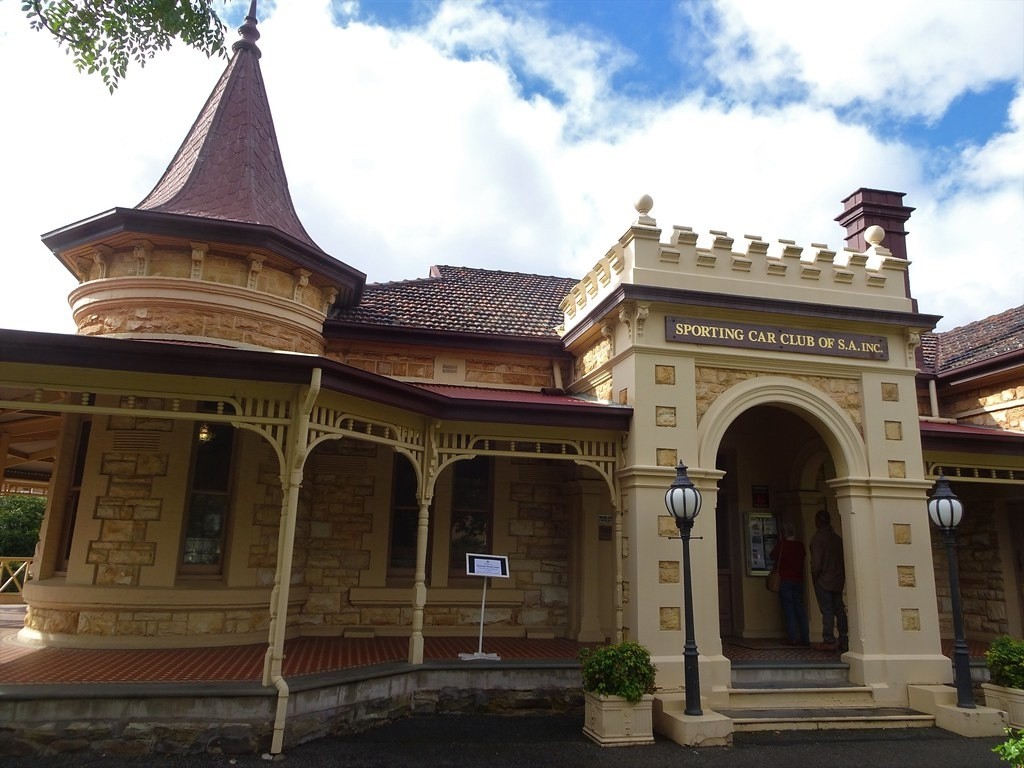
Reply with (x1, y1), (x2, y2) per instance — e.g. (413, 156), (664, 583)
(771, 522), (810, 645)
(810, 511), (848, 651)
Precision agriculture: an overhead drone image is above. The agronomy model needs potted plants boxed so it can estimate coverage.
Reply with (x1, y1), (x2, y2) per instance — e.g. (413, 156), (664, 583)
(982, 634), (1023, 733)
(578, 642), (658, 747)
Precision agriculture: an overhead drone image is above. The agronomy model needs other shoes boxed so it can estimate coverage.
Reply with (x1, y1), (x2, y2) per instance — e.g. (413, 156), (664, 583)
(780, 639), (810, 647)
(812, 636), (848, 653)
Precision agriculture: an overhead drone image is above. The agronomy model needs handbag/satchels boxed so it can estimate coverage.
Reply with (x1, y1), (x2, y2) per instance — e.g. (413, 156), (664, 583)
(766, 571), (781, 592)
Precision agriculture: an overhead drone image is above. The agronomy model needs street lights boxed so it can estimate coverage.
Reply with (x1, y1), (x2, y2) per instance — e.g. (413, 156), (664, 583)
(927, 474), (976, 708)
(664, 457), (705, 717)
